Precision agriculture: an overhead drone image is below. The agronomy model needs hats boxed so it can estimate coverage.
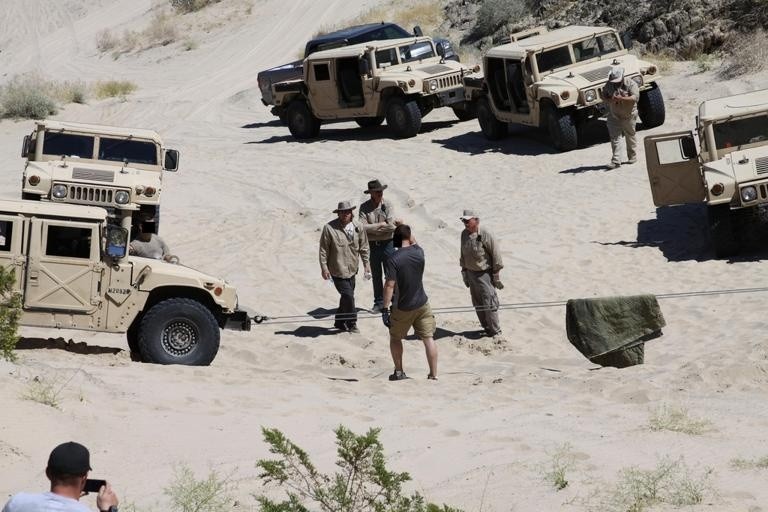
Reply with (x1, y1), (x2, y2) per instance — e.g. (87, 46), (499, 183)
(607, 67), (624, 84)
(47, 441), (93, 478)
(363, 180), (388, 195)
(370, 302), (384, 315)
(460, 209), (479, 220)
(332, 201), (356, 214)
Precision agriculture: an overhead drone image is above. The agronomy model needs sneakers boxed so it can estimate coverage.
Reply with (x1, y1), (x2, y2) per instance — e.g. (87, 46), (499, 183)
(333, 321), (362, 335)
(606, 162), (621, 169)
(627, 157), (638, 164)
(388, 369), (407, 381)
(479, 327), (502, 341)
(427, 374), (438, 381)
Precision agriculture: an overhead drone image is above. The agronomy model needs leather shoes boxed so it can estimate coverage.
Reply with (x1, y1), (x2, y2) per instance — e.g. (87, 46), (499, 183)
(370, 240), (391, 245)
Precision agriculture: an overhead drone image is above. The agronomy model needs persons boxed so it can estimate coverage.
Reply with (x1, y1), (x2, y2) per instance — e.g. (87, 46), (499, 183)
(129, 213), (174, 263)
(358, 181), (396, 313)
(318, 201), (369, 333)
(382, 219), (440, 381)
(600, 67), (640, 171)
(1, 442), (120, 511)
(458, 210), (504, 339)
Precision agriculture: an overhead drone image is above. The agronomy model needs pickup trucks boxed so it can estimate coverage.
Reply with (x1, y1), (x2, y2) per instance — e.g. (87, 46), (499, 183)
(256, 21), (461, 124)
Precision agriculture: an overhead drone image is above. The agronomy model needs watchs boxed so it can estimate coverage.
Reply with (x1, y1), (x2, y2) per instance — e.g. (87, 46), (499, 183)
(100, 505), (119, 512)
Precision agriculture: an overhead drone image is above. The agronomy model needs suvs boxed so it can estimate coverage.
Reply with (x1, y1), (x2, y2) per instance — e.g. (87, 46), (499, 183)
(643, 89), (768, 253)
(477, 24), (665, 151)
(0, 200), (251, 366)
(272, 36), (480, 139)
(20, 119), (179, 236)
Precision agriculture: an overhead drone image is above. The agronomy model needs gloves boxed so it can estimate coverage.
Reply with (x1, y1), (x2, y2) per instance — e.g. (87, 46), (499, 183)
(493, 276), (503, 290)
(460, 271), (470, 288)
(380, 305), (394, 329)
